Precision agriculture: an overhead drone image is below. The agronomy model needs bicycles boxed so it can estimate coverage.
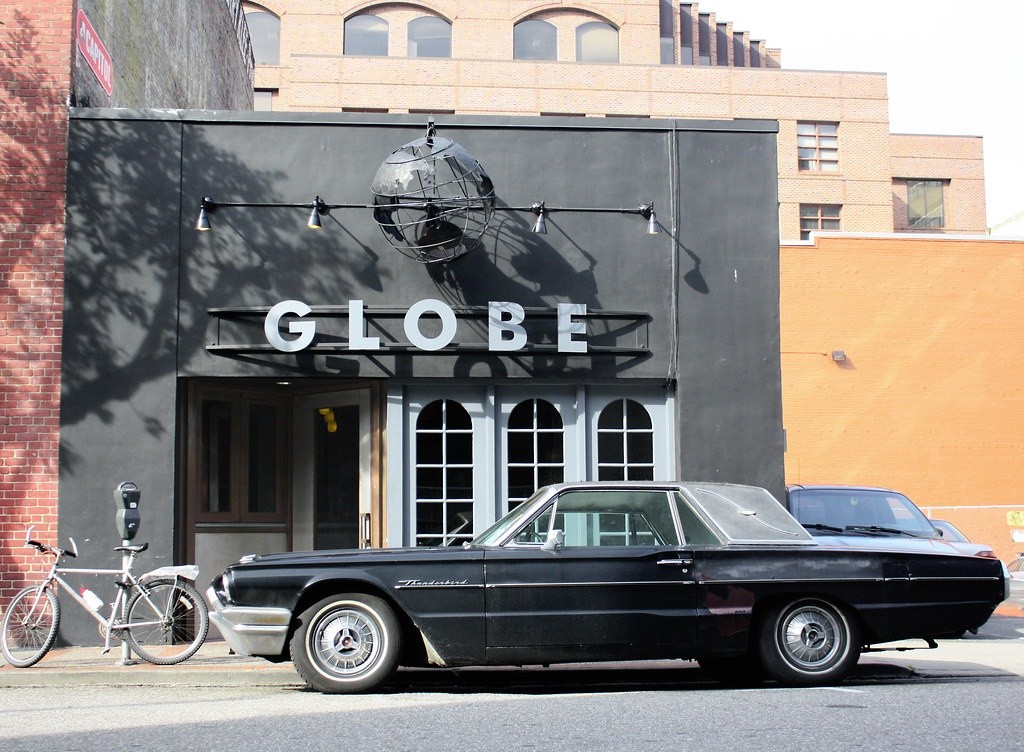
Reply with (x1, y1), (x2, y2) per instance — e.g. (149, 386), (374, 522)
(1, 524), (209, 666)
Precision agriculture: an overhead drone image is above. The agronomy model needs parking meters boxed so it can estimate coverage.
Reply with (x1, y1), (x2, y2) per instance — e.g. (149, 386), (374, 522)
(113, 480), (143, 667)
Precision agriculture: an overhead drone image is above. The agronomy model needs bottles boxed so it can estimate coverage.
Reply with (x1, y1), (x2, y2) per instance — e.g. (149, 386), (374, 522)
(79, 587), (104, 611)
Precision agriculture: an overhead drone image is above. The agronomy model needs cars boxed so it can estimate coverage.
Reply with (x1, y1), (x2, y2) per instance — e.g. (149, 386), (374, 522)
(207, 480), (1004, 692)
(930, 518), (970, 542)
(785, 485), (945, 542)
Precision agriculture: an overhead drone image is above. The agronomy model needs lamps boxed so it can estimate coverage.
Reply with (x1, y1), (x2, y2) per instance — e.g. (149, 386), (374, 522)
(319, 408), (337, 433)
(193, 194), (663, 236)
(832, 350), (846, 362)
